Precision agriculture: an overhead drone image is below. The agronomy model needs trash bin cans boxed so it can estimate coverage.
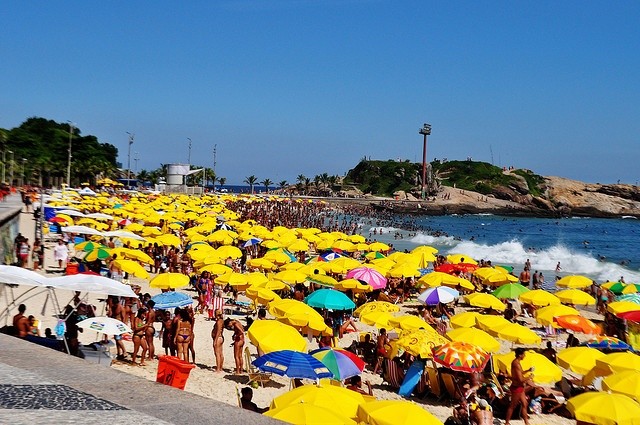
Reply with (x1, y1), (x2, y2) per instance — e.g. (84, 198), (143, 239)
(66, 263), (79, 275)
(157, 355), (194, 389)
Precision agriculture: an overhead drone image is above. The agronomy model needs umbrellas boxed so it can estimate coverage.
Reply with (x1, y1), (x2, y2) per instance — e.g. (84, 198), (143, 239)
(431, 341), (490, 373)
(606, 351), (640, 370)
(248, 319), (310, 355)
(566, 392), (640, 425)
(555, 347), (606, 373)
(250, 351), (333, 389)
(493, 349), (563, 385)
(21, 178), (99, 213)
(97, 177), (238, 222)
(262, 384), (442, 424)
(189, 218), (436, 318)
(603, 370), (640, 400)
(62, 210), (191, 279)
(75, 316), (132, 364)
(421, 253), (640, 347)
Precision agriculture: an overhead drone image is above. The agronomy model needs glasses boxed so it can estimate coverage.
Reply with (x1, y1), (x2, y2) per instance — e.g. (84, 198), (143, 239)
(31, 319), (35, 322)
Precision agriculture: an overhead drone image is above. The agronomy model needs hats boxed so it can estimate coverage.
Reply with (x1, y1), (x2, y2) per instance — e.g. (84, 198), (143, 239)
(478, 399), (488, 408)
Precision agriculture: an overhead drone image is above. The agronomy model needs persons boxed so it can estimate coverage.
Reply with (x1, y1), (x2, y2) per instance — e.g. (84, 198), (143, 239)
(453, 396), (471, 424)
(452, 234), (477, 242)
(119, 281), (141, 330)
(240, 388), (269, 413)
(505, 346), (535, 425)
(212, 308), (225, 373)
(132, 308), (148, 366)
(145, 300), (156, 361)
(13, 304), (30, 337)
(323, 322), (416, 394)
(13, 215), (67, 272)
(45, 328), (57, 338)
(28, 315), (40, 336)
(347, 194), (445, 236)
(228, 191), (346, 232)
(74, 291), (95, 317)
(227, 318), (246, 375)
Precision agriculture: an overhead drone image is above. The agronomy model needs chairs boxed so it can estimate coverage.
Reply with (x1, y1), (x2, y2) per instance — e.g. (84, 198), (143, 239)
(235, 384), (241, 407)
(422, 366), (447, 402)
(359, 332), (372, 355)
(212, 297), (224, 320)
(244, 347), (272, 388)
(387, 359), (400, 389)
(440, 373), (463, 404)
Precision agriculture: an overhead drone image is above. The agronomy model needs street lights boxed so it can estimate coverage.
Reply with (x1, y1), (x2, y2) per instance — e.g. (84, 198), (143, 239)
(187, 138), (192, 164)
(125, 130), (136, 186)
(66, 119), (78, 188)
(213, 144), (218, 173)
(134, 152), (140, 173)
